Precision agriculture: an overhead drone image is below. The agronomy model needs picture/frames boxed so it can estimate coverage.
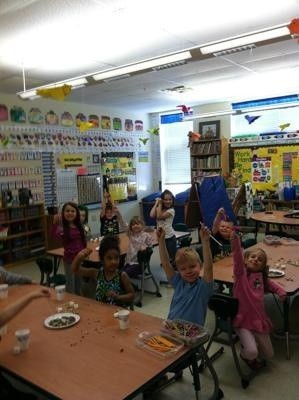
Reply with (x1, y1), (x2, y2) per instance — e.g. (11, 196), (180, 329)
(199, 120), (220, 139)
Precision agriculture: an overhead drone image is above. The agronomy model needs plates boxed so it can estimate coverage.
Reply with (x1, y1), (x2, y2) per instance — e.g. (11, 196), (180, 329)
(278, 237), (297, 245)
(264, 235), (281, 245)
(135, 329), (184, 358)
(267, 268), (286, 278)
(43, 312), (81, 329)
(159, 318), (209, 348)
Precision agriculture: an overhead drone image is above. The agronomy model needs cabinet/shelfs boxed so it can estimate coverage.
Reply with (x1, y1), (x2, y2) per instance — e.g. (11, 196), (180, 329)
(189, 139), (230, 184)
(0, 203), (48, 266)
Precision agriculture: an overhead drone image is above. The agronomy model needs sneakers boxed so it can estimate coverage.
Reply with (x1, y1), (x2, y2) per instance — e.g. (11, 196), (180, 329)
(239, 353), (263, 370)
(81, 276), (90, 286)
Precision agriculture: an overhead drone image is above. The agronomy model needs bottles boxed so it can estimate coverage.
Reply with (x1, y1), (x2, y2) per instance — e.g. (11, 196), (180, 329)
(283, 181), (292, 202)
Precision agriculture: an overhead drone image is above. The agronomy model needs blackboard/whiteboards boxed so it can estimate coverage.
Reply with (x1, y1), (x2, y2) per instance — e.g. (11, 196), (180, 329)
(228, 139), (298, 196)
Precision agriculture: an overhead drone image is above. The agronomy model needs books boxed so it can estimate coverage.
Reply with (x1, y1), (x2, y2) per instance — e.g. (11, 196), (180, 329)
(191, 140), (220, 183)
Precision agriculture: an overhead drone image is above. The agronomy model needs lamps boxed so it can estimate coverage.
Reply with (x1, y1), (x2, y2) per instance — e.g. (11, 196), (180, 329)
(12, 17), (298, 102)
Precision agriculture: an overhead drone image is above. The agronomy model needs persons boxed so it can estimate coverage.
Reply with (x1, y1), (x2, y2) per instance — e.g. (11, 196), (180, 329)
(77, 204), (92, 237)
(112, 205), (155, 277)
(149, 189), (178, 272)
(71, 235), (135, 311)
(50, 202), (87, 297)
(0, 287), (52, 329)
(100, 192), (120, 236)
(155, 225), (214, 328)
(0, 266), (38, 285)
(229, 230), (287, 371)
(211, 207), (245, 295)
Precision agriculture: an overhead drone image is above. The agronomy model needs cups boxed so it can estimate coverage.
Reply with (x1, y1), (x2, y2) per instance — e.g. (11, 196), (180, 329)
(54, 285), (66, 302)
(14, 329), (31, 352)
(0, 284), (9, 300)
(119, 309), (130, 330)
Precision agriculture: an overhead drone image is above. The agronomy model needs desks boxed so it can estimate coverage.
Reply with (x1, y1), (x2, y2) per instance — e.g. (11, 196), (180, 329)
(249, 209), (299, 238)
(198, 233), (299, 360)
(0, 284), (210, 400)
(46, 227), (191, 276)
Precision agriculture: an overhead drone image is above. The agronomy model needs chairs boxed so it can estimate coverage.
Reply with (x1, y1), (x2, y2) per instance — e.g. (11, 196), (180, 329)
(158, 238), (192, 285)
(34, 257), (66, 289)
(117, 281), (138, 311)
(259, 198), (299, 210)
(130, 247), (162, 308)
(271, 291), (299, 361)
(173, 223), (188, 246)
(200, 292), (260, 390)
(242, 238), (256, 248)
